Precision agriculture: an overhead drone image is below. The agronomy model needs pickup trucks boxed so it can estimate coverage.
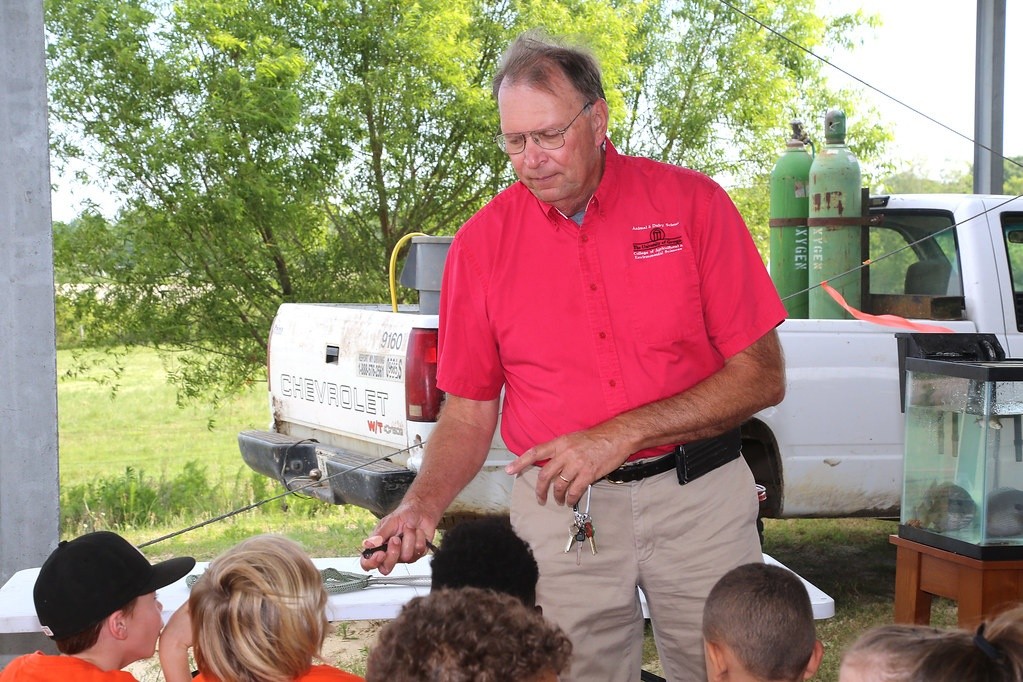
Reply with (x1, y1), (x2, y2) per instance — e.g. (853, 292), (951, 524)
(236, 193), (1022, 549)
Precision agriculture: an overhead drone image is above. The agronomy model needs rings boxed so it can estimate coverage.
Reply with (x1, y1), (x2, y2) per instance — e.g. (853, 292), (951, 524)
(558, 474), (571, 483)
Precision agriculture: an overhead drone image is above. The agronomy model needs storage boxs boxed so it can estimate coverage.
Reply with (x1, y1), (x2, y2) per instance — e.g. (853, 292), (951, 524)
(898, 356), (1023, 563)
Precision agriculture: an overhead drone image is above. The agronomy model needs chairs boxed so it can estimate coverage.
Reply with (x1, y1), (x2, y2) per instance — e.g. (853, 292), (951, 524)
(904, 257), (951, 296)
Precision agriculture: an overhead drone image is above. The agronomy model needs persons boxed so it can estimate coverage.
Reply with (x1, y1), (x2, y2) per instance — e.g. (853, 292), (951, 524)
(190, 535), (368, 682)
(702, 562), (824, 682)
(0, 531), (196, 682)
(360, 33), (788, 682)
(429, 520), (543, 615)
(839, 604), (1023, 682)
(365, 588), (573, 682)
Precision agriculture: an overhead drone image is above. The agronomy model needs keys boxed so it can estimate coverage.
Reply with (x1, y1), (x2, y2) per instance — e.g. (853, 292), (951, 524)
(564, 523), (597, 564)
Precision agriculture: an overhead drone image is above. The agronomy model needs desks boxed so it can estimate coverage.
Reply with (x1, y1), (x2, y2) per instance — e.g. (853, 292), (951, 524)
(0, 552), (836, 682)
(888, 534), (1023, 631)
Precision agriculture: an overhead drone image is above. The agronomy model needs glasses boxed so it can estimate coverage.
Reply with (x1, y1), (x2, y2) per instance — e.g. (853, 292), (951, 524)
(493, 102), (592, 155)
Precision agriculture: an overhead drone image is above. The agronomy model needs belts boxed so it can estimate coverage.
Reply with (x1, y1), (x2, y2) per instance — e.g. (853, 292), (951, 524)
(602, 453), (676, 484)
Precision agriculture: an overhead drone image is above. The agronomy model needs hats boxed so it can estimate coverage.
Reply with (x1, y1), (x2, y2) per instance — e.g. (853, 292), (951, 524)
(33, 531), (195, 641)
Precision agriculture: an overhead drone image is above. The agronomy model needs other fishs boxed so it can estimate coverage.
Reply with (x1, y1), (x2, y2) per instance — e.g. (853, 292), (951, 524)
(912, 479), (977, 533)
(986, 487), (1023, 538)
(974, 416), (1002, 430)
(362, 536), (439, 559)
